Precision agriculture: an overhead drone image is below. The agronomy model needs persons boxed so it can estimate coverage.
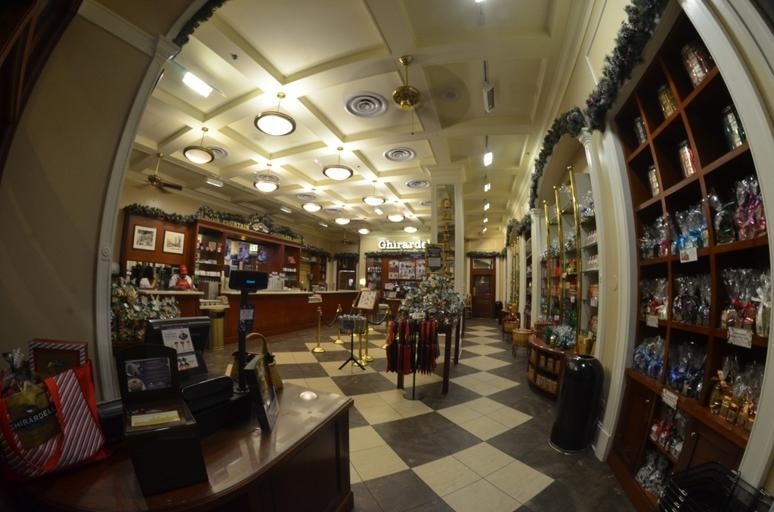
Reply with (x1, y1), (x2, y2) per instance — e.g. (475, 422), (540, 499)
(166, 263), (195, 290)
(137, 265), (158, 290)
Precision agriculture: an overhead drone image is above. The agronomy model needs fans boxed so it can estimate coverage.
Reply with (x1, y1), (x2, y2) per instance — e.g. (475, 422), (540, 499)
(130, 153), (182, 194)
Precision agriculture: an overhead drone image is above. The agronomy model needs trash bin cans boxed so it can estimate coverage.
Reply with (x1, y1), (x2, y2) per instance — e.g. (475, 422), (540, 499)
(547, 352), (605, 459)
(493, 300), (503, 319)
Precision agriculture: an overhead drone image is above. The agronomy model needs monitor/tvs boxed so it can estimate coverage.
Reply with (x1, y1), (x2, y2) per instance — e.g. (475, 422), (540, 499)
(143, 315), (212, 382)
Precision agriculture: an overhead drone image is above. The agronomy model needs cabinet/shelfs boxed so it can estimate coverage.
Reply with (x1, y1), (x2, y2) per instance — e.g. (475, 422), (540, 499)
(195, 226), (298, 287)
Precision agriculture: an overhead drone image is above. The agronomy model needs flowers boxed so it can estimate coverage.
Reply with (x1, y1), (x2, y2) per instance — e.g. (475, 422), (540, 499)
(401, 274), (461, 315)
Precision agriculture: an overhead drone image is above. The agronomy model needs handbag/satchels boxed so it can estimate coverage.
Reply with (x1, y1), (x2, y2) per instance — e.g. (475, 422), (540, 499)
(0, 359), (107, 485)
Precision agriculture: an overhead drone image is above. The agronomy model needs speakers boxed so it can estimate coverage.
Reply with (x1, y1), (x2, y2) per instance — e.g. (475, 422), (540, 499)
(481, 83), (495, 112)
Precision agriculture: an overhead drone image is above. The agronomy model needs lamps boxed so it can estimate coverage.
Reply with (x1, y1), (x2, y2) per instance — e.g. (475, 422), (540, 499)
(181, 94), (352, 214)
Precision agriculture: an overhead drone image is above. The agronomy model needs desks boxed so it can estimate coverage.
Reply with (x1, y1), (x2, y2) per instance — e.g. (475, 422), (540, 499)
(397, 311), (461, 394)
